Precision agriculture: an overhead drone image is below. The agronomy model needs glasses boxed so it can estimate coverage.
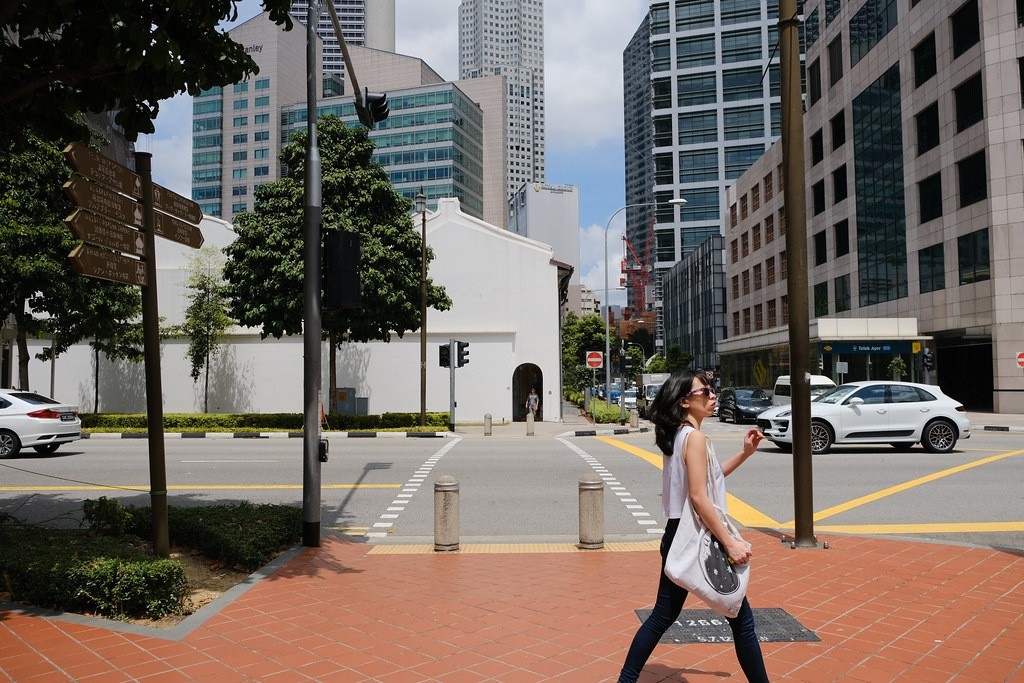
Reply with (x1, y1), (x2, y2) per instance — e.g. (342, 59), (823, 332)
(686, 387), (715, 397)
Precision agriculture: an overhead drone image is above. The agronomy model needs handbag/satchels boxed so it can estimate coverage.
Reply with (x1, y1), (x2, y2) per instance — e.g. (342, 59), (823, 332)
(665, 431), (749, 618)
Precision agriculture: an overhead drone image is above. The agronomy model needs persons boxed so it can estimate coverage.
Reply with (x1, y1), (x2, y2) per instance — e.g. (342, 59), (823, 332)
(617, 368), (770, 683)
(525, 387), (539, 416)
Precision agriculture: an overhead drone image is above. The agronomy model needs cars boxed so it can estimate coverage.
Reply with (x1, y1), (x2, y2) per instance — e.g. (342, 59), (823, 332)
(718, 385), (774, 423)
(758, 381), (972, 454)
(712, 399), (719, 414)
(0, 388), (82, 458)
(591, 383), (622, 404)
(617, 390), (637, 411)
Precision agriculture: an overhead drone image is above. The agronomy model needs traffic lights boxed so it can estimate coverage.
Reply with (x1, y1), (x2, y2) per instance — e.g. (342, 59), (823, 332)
(458, 341), (470, 367)
(439, 345), (450, 367)
(819, 355), (823, 369)
(924, 352), (935, 372)
(362, 86), (390, 127)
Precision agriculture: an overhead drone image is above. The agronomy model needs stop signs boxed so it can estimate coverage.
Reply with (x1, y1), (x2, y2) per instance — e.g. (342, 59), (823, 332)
(1016, 352), (1024, 369)
(586, 351), (603, 368)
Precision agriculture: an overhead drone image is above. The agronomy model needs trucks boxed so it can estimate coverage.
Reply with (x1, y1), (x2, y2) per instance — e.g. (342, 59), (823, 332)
(636, 383), (664, 417)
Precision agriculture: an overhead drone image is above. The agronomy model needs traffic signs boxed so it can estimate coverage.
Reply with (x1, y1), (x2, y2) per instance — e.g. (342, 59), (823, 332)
(64, 142), (204, 287)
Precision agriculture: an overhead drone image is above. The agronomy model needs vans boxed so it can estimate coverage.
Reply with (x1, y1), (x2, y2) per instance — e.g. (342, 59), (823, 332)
(773, 375), (837, 408)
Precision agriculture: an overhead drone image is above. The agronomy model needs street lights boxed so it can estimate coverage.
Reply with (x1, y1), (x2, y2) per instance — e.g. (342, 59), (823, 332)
(583, 287), (626, 318)
(416, 186), (428, 426)
(605, 199), (688, 407)
(638, 321), (666, 359)
(628, 342), (645, 373)
